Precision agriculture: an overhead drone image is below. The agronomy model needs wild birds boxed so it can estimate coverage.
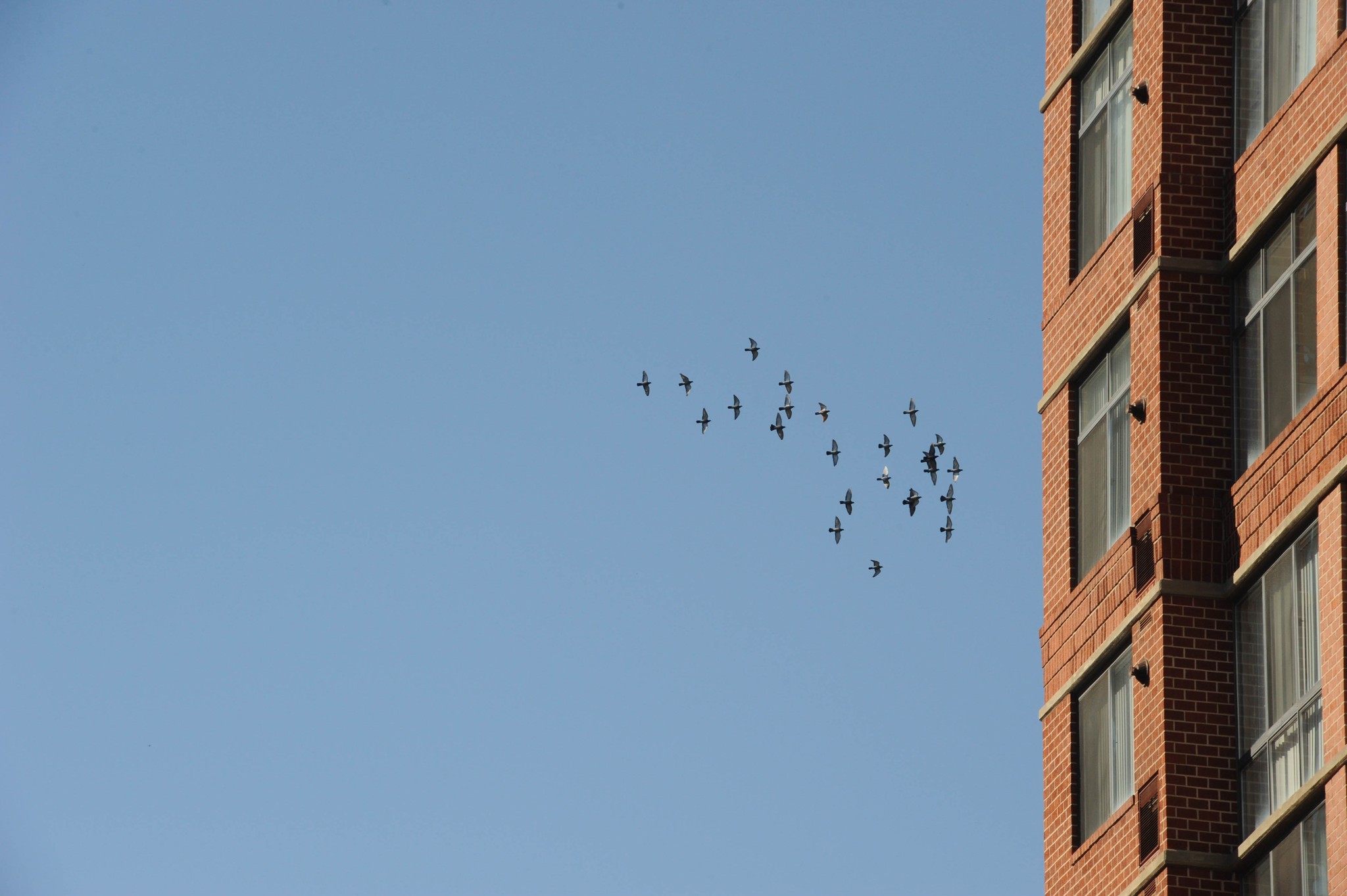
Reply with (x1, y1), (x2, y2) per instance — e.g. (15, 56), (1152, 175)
(941, 485), (956, 515)
(637, 371), (652, 397)
(814, 402), (830, 423)
(868, 559), (883, 577)
(903, 488), (921, 517)
(744, 337), (760, 361)
(947, 457), (962, 483)
(921, 442), (940, 487)
(679, 372), (694, 396)
(778, 395), (796, 419)
(840, 487), (854, 516)
(933, 434), (946, 456)
(826, 439), (841, 467)
(903, 397), (920, 428)
(876, 465), (892, 489)
(770, 413), (786, 440)
(728, 394), (743, 420)
(779, 368), (794, 393)
(828, 515), (845, 544)
(696, 407), (713, 434)
(938, 515), (955, 543)
